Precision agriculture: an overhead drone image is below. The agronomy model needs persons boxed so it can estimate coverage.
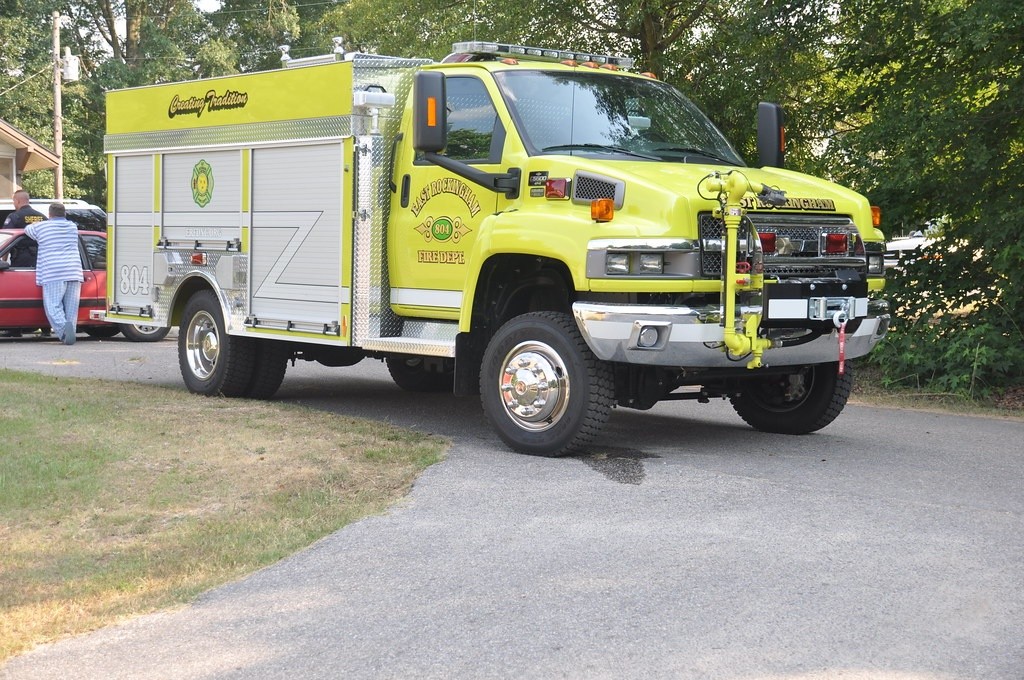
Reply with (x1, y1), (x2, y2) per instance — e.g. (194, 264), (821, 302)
(0, 190), (51, 337)
(24, 202), (84, 345)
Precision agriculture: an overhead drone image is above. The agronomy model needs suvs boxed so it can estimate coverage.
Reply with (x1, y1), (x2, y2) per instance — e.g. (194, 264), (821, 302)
(0, 195), (109, 237)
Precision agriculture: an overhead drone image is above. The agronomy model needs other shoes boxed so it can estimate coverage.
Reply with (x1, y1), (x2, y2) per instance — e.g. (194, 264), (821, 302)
(62, 321), (77, 346)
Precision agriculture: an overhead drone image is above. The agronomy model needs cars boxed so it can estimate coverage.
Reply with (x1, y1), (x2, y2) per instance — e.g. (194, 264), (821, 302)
(884, 210), (982, 276)
(0, 229), (173, 341)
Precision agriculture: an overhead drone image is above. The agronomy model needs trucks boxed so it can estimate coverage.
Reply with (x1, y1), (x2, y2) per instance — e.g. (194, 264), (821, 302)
(85, 39), (896, 458)
